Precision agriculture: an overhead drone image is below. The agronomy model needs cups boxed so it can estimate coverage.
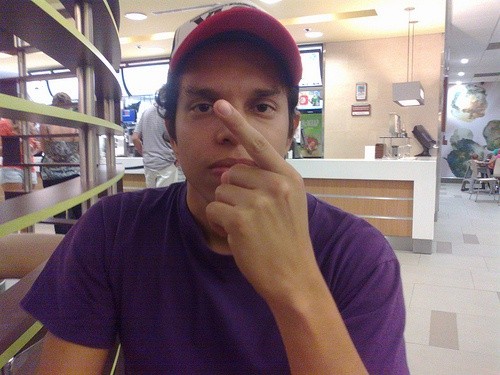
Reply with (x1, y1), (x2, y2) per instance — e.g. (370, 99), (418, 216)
(392, 145), (398, 156)
(300, 96), (308, 105)
(376, 144), (383, 158)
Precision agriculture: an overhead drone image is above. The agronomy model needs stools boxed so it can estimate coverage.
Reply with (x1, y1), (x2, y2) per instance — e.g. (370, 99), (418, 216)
(475, 177), (497, 201)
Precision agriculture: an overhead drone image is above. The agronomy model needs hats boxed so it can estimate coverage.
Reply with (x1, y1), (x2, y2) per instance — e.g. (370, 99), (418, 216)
(164, 3), (303, 90)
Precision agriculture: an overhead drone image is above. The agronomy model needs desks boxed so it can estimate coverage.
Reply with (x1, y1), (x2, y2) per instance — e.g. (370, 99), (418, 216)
(460, 161), (491, 194)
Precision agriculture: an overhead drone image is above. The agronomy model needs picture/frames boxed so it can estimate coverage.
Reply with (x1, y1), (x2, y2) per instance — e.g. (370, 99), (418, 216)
(356, 83), (367, 99)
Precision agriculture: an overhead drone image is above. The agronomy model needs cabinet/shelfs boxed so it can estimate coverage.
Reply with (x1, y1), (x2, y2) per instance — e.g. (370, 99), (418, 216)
(0, 0), (128, 375)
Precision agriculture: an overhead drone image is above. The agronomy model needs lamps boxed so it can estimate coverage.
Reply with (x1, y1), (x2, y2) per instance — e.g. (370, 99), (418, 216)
(391, 7), (425, 107)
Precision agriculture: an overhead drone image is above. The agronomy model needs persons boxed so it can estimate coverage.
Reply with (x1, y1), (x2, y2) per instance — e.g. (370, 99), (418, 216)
(0, 93), (179, 234)
(21, 3), (413, 375)
